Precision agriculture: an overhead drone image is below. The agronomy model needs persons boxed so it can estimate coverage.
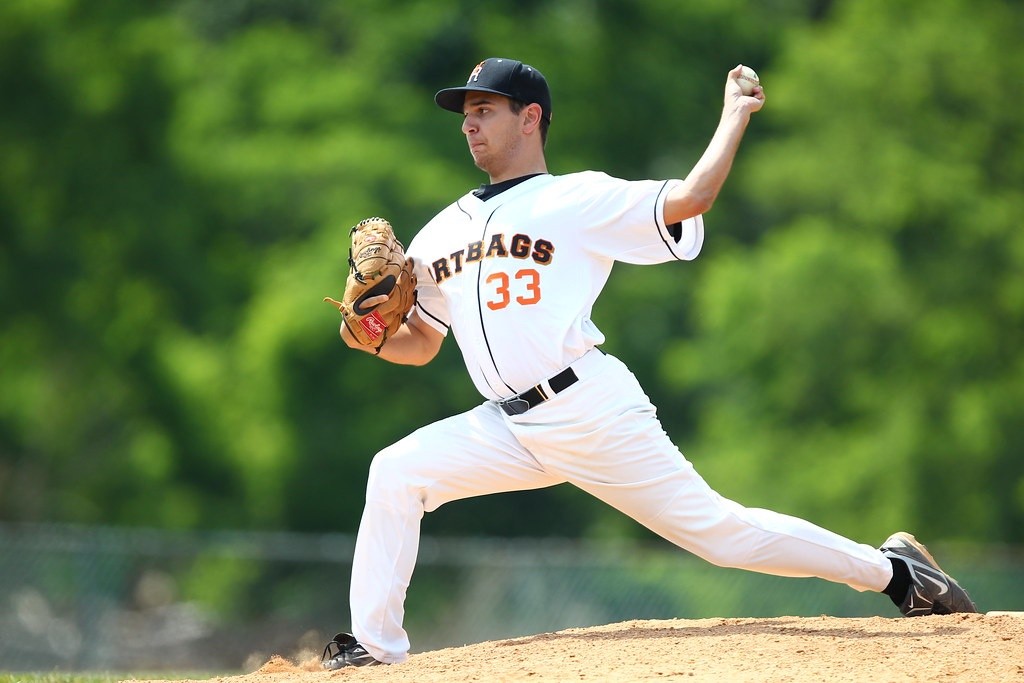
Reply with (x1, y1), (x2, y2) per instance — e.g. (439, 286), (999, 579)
(306, 55), (980, 671)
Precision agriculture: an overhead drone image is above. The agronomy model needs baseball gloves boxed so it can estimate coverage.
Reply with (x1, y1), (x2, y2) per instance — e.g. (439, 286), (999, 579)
(336, 216), (418, 351)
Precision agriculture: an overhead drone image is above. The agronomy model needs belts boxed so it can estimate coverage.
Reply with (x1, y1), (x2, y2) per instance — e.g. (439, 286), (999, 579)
(498, 345), (606, 416)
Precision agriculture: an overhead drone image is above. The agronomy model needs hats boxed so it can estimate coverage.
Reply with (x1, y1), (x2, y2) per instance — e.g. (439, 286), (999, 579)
(434, 58), (552, 121)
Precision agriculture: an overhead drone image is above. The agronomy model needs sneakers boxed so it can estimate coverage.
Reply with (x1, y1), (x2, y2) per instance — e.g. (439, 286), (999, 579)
(321, 632), (391, 672)
(878, 531), (978, 617)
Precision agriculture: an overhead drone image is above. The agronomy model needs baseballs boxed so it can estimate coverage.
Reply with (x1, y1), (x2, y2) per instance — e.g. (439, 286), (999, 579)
(733, 64), (762, 95)
